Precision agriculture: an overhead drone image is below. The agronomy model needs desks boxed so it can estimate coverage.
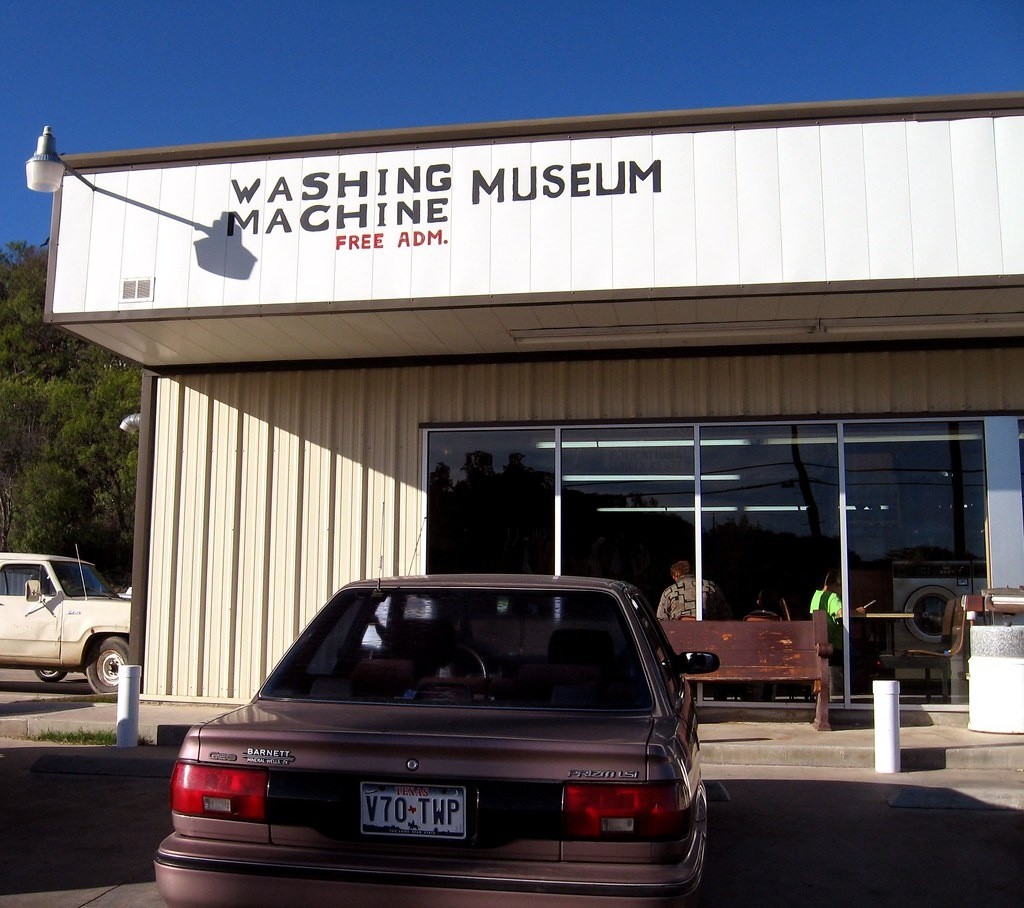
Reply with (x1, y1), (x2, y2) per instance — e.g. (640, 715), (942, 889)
(851, 614), (914, 705)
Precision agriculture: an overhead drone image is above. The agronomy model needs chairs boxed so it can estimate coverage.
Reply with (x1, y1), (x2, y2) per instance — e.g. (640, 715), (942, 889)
(878, 597), (967, 704)
(311, 615), (620, 703)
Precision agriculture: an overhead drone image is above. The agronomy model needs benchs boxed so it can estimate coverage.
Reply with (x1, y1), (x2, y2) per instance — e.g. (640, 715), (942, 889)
(648, 610), (834, 730)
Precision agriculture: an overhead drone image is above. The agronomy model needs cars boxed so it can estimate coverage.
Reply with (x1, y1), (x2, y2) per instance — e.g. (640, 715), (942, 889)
(0, 552), (133, 694)
(152, 574), (720, 908)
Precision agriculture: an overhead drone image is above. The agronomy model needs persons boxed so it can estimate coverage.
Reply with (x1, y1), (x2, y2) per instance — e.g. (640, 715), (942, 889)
(809, 569), (882, 704)
(656, 560), (783, 702)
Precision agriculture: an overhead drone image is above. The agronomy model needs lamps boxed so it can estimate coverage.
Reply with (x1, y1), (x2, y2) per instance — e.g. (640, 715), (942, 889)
(24, 127), (97, 192)
